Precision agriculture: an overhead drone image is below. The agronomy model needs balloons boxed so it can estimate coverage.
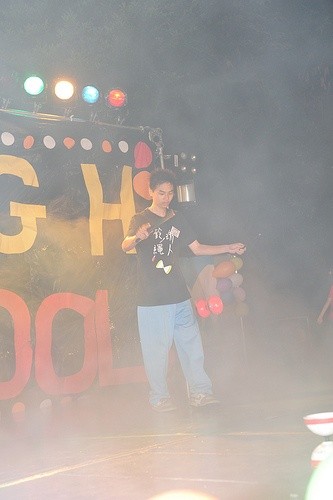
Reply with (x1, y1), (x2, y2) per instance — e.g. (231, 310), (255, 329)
(212, 258), (245, 308)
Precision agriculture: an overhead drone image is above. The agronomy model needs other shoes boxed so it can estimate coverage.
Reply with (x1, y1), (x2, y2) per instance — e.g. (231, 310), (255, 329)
(154, 401), (176, 413)
(189, 393), (220, 408)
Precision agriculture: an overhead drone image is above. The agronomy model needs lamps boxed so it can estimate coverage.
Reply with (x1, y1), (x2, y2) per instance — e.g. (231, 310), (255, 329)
(24, 77), (132, 126)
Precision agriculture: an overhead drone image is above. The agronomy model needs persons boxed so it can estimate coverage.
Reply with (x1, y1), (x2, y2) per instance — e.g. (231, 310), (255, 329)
(122, 169), (246, 412)
(316, 285), (333, 324)
